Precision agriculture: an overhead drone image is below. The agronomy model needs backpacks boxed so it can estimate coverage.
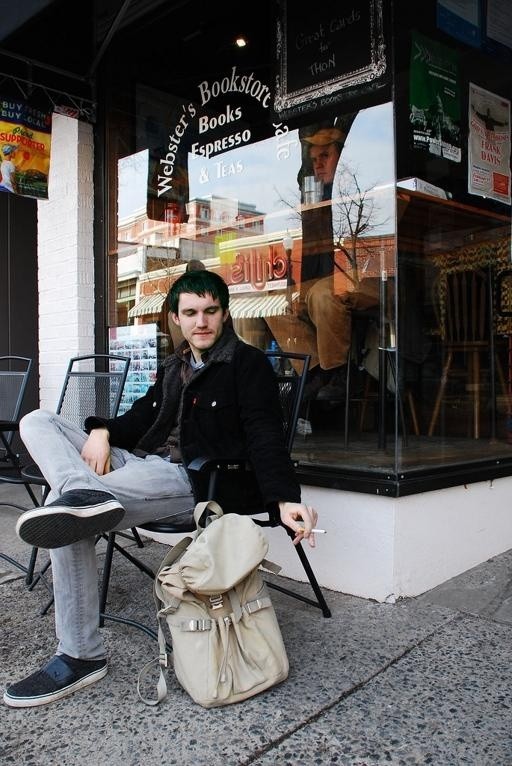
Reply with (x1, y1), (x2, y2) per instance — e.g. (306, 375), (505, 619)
(135, 497), (291, 710)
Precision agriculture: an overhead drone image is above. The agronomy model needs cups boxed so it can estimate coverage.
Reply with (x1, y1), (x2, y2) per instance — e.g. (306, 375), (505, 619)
(305, 177), (325, 202)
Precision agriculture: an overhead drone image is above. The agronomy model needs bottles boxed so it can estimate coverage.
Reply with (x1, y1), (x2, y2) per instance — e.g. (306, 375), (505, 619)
(164, 201), (181, 237)
(264, 341), (280, 375)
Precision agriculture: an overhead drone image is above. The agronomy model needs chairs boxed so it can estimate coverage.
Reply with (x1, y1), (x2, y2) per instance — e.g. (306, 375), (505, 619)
(98, 353), (332, 627)
(1, 354), (155, 616)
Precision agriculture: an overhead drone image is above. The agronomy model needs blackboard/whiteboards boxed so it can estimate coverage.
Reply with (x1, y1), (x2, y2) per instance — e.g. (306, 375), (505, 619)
(274, 2), (386, 114)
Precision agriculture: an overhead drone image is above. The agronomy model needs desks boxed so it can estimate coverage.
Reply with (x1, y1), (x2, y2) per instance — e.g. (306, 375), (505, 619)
(104, 184), (510, 474)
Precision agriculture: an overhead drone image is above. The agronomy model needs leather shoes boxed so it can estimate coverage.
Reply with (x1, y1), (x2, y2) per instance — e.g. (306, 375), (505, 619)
(302, 368), (330, 403)
(315, 363), (361, 402)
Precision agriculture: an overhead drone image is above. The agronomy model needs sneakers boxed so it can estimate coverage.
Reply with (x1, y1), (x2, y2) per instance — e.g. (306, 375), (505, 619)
(15, 490), (126, 549)
(2, 652), (109, 708)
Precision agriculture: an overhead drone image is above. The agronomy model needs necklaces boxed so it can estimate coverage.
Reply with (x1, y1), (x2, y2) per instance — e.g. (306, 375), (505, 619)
(190, 348), (208, 369)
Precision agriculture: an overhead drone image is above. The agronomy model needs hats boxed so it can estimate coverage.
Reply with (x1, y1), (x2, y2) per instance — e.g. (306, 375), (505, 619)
(3, 144), (17, 157)
(301, 128), (349, 151)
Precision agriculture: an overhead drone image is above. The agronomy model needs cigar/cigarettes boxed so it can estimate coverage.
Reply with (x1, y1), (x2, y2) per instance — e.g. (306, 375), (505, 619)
(300, 528), (326, 534)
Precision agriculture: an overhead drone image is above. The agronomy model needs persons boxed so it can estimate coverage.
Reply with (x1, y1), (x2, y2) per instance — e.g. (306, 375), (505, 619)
(262, 129), (394, 401)
(3, 269), (319, 709)
(167, 259), (206, 352)
(472, 103), (509, 131)
(0, 144), (19, 194)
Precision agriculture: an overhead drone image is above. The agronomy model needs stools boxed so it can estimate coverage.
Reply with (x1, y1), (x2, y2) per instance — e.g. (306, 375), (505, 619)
(301, 305), (410, 449)
(428, 339), (510, 441)
